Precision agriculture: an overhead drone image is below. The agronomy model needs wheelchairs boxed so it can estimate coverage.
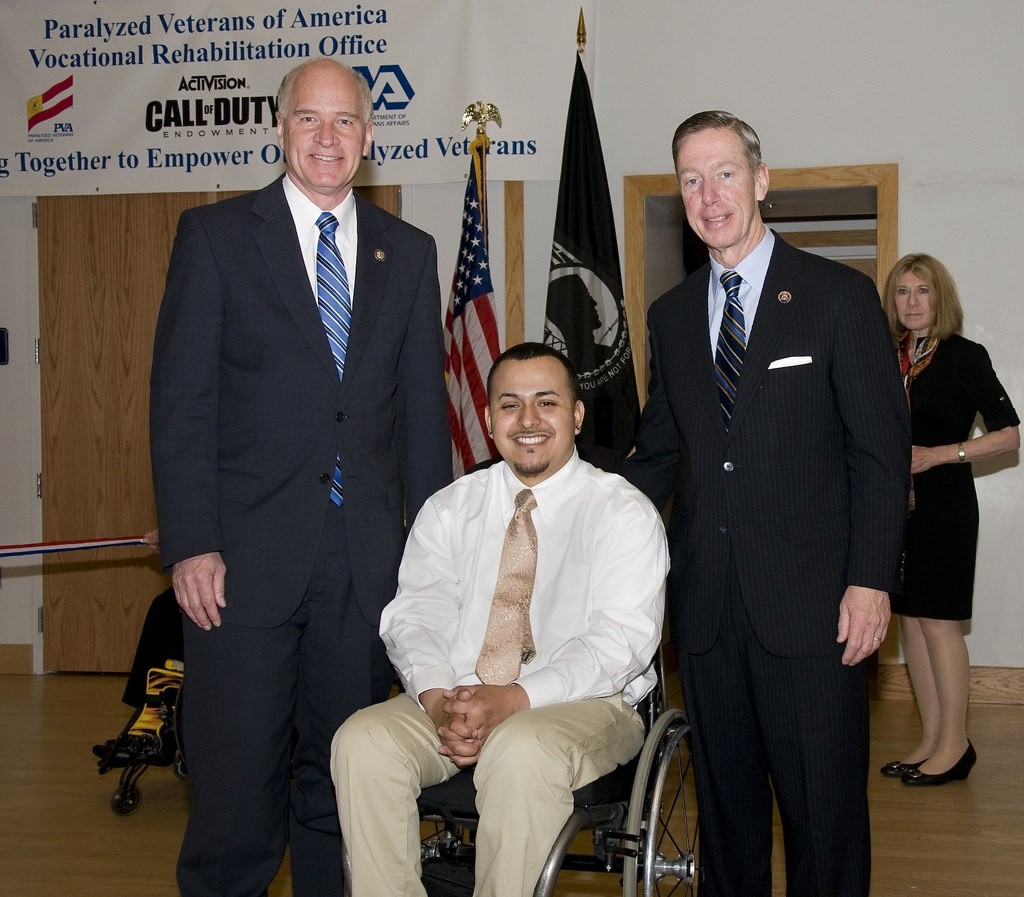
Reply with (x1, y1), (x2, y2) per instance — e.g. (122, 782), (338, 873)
(97, 659), (184, 817)
(417, 635), (706, 897)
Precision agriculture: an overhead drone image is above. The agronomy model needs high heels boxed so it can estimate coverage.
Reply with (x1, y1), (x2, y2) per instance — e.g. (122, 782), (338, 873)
(901, 738), (977, 786)
(880, 757), (930, 776)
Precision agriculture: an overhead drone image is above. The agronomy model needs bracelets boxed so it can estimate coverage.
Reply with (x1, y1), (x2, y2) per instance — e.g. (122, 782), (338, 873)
(958, 442), (965, 464)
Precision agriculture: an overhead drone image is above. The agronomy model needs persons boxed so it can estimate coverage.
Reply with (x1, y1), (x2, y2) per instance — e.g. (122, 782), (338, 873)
(93, 529), (185, 766)
(443, 133), (501, 479)
(883, 254), (1020, 787)
(151, 57), (455, 897)
(619, 110), (912, 897)
(329, 342), (671, 896)
(544, 55), (639, 468)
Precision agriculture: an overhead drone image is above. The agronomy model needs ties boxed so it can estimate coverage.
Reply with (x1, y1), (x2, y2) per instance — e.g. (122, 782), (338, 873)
(712, 270), (746, 439)
(317, 211), (352, 508)
(475, 489), (538, 685)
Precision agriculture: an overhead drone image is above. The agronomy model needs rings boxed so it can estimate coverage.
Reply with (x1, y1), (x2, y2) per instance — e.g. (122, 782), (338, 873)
(874, 638), (882, 640)
